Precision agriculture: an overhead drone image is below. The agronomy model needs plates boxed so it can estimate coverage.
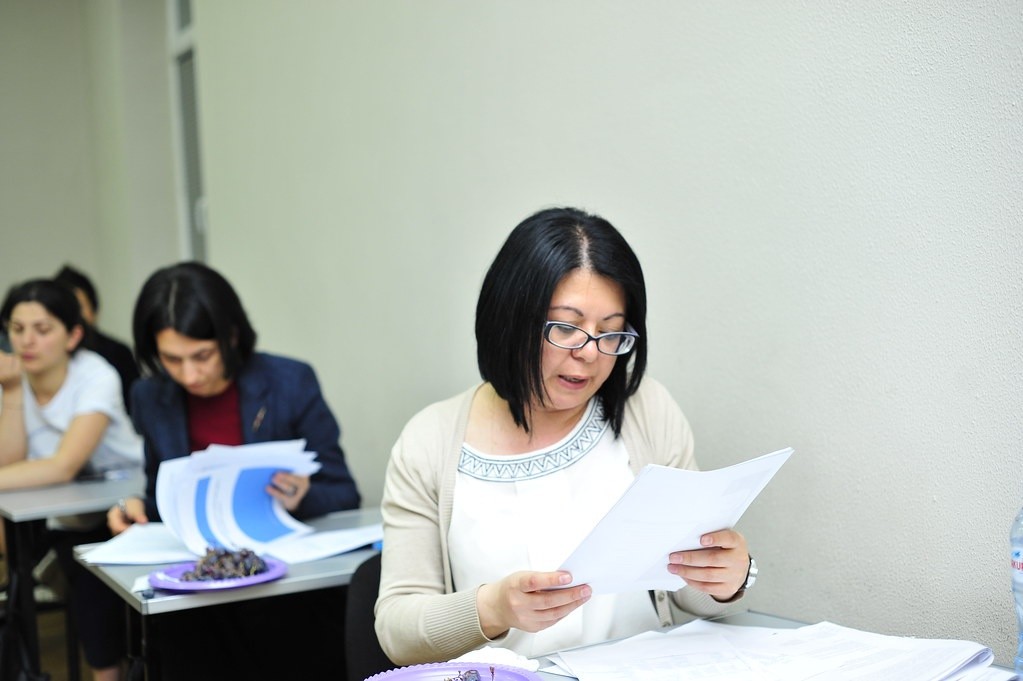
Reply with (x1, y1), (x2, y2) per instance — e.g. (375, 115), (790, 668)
(149, 555), (286, 590)
(363, 662), (542, 681)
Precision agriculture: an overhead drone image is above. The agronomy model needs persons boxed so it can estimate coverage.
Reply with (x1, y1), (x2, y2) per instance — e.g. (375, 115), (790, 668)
(374, 206), (763, 667)
(0, 261), (359, 681)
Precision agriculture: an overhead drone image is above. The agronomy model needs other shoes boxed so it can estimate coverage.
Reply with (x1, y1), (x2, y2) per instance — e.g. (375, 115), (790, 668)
(32, 582), (60, 602)
(0, 551), (8, 600)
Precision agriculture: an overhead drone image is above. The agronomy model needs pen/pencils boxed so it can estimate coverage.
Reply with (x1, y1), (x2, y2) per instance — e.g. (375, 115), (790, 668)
(118, 499), (130, 525)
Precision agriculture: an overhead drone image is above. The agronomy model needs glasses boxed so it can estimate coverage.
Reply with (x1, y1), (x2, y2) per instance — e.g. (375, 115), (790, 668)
(543, 317), (641, 357)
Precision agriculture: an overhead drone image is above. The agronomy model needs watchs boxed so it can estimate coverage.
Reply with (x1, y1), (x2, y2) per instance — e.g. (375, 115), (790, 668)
(740, 555), (758, 591)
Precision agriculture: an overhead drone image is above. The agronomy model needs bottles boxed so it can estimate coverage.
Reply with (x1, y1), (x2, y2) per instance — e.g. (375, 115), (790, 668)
(1008, 506), (1023, 680)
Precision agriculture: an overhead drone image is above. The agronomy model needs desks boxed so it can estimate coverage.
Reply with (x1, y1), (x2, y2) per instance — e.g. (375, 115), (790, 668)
(0, 479), (1016, 681)
